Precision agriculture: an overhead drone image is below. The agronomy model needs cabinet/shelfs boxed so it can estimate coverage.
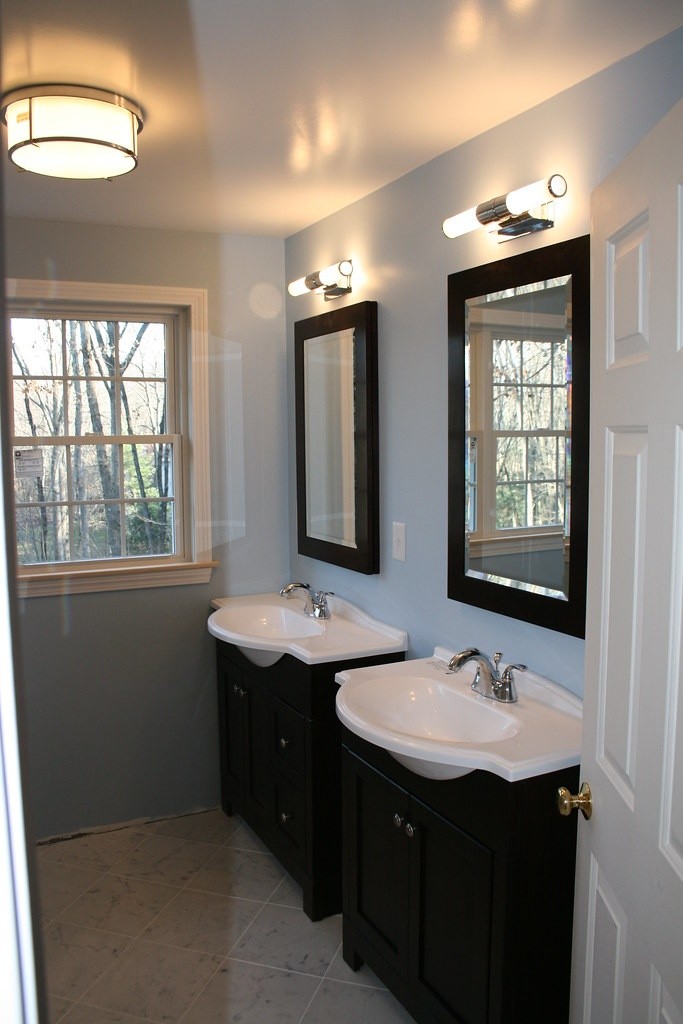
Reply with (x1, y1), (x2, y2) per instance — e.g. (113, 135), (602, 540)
(211, 608), (406, 924)
(339, 718), (581, 1024)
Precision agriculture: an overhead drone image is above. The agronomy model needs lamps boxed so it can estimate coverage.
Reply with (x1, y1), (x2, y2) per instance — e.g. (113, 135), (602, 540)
(288, 260), (353, 301)
(441, 175), (566, 243)
(1, 84), (143, 178)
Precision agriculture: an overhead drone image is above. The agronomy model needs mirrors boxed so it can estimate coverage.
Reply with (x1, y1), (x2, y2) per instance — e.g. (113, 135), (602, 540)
(295, 300), (378, 575)
(448, 233), (592, 640)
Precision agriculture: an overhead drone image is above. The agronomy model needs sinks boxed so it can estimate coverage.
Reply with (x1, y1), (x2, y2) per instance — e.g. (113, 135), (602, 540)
(207, 603), (328, 668)
(336, 673), (527, 780)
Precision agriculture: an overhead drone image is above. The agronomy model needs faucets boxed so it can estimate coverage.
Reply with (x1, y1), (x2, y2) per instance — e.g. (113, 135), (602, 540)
(447, 648), (499, 696)
(280, 581), (319, 615)
